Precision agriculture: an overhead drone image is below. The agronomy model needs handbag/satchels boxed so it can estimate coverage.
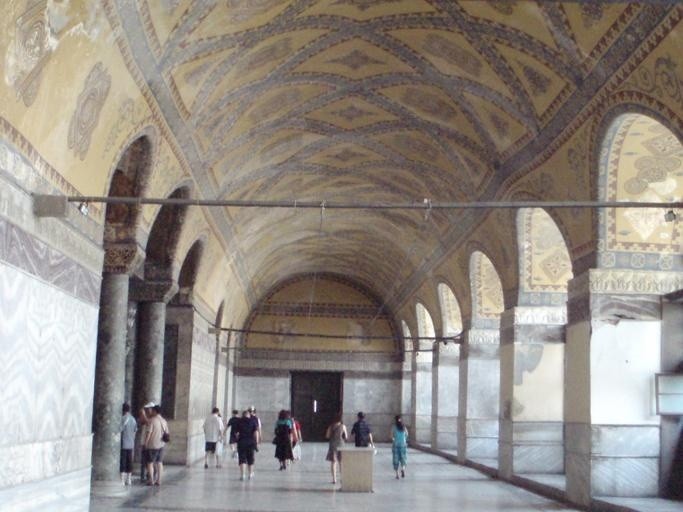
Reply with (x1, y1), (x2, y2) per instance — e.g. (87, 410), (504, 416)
(163, 432), (169, 442)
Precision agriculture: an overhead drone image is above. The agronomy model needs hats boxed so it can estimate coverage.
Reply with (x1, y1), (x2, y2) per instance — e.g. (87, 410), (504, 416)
(144, 402), (155, 408)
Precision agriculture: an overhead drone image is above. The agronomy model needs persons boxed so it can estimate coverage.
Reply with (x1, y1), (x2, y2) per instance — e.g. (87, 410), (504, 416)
(325, 416), (348, 484)
(390, 416), (408, 478)
(349, 412), (376, 447)
(203, 406), (262, 480)
(121, 402), (169, 485)
(275, 411), (302, 470)
